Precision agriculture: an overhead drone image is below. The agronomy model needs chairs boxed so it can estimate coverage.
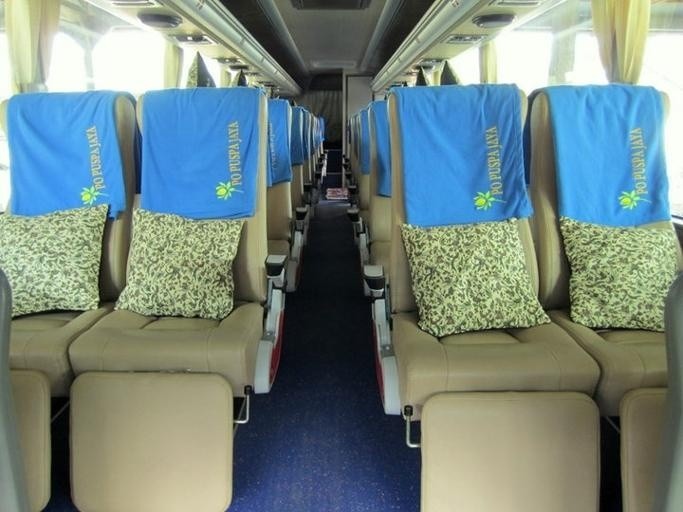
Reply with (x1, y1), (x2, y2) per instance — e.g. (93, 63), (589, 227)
(362, 83), (601, 511)
(67, 84), (290, 511)
(1, 89), (143, 511)
(523, 83), (683, 512)
(88, 83), (329, 294)
(342, 100), (392, 299)
